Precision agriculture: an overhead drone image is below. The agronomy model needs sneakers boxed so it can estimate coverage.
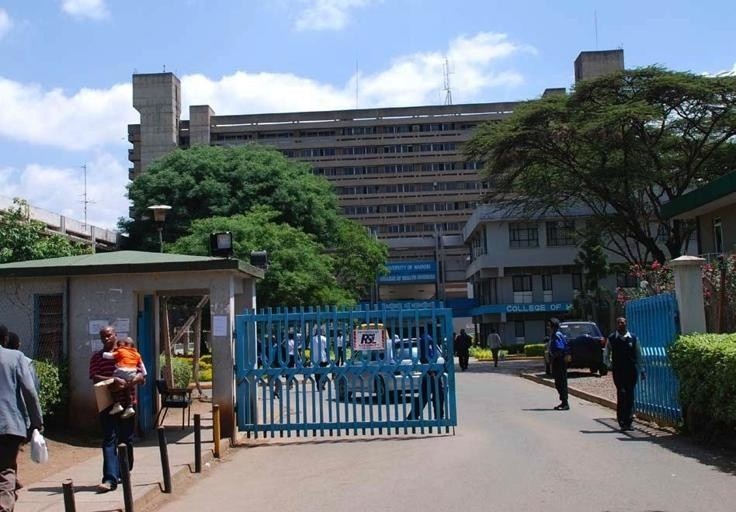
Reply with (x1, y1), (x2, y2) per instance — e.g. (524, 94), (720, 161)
(554, 402), (570, 411)
(120, 407), (136, 420)
(108, 404), (125, 416)
(95, 483), (112, 493)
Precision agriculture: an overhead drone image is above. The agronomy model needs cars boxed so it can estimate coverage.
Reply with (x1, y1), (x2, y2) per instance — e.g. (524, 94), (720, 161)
(335, 337), (449, 403)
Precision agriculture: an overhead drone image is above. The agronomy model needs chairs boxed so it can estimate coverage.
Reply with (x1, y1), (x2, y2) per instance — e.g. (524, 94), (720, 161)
(152, 379), (193, 429)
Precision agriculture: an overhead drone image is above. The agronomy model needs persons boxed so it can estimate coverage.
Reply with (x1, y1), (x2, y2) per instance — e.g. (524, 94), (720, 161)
(547, 316), (575, 410)
(0, 324), (46, 511)
(7, 330), (40, 490)
(603, 316), (649, 432)
(258, 318), (505, 421)
(88, 326), (137, 493)
(98, 336), (148, 419)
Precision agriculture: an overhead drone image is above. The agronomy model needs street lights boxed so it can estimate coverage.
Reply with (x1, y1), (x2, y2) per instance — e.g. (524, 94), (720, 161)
(148, 204), (172, 252)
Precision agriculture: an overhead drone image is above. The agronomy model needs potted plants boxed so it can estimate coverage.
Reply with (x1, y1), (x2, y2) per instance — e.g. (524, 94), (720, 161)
(30, 358), (62, 437)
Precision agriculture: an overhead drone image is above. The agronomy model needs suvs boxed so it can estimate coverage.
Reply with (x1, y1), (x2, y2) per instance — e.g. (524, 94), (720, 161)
(543, 322), (607, 377)
